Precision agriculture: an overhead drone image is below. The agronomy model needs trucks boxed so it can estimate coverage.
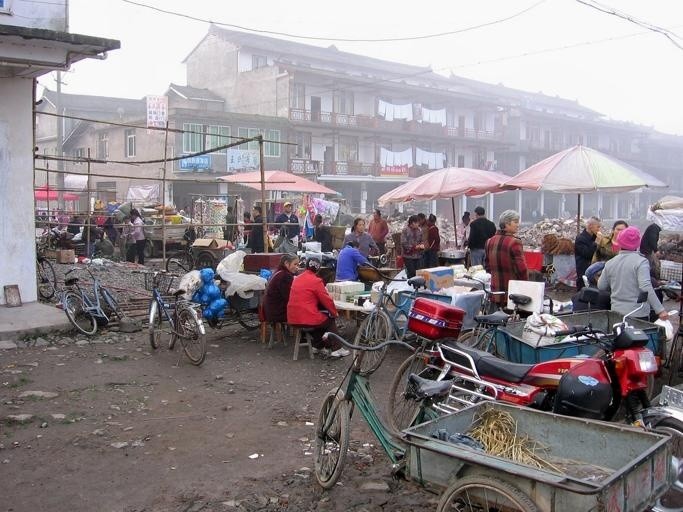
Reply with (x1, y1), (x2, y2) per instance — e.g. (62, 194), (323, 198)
(140, 224), (196, 258)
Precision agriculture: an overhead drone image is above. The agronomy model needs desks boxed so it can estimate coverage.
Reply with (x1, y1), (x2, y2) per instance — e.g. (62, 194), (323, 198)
(333, 298), (376, 319)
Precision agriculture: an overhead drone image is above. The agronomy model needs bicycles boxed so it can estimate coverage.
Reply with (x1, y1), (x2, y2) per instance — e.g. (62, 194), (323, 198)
(35, 248), (58, 300)
(60, 251), (126, 338)
(128, 265), (207, 368)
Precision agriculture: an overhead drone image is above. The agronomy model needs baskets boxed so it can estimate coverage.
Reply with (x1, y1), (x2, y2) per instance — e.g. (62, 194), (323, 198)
(145, 273), (172, 292)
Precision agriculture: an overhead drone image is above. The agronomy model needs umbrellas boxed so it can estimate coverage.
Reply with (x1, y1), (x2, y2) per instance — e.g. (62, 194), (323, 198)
(375, 165), (522, 249)
(498, 143), (669, 236)
(215, 170), (337, 206)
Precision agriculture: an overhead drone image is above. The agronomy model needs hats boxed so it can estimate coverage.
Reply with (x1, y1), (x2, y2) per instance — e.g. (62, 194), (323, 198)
(284, 202), (292, 206)
(617, 227), (641, 250)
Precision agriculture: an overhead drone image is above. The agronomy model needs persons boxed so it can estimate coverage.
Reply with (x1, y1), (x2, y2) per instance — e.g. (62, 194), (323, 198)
(460, 206), (497, 267)
(262, 254), (310, 345)
(481, 210), (529, 313)
(575, 216), (668, 322)
(367, 209), (387, 254)
(400, 214), (441, 285)
(41, 209), (144, 264)
(286, 258), (353, 357)
(223, 202), (333, 253)
(180, 206), (190, 217)
(335, 219), (377, 282)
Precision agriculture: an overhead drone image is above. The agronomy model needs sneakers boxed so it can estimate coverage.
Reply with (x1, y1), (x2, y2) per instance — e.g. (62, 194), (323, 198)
(312, 347), (329, 354)
(331, 348), (350, 357)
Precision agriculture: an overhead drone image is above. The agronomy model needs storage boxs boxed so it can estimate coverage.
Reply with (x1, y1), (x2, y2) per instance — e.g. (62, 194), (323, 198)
(192, 239), (232, 248)
(56, 249), (76, 264)
(416, 267), (454, 290)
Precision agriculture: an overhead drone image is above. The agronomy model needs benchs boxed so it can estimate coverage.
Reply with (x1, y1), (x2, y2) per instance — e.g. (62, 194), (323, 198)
(261, 319), (315, 360)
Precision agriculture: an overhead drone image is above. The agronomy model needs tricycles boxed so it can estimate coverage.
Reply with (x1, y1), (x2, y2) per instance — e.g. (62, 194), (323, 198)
(164, 229), (328, 331)
